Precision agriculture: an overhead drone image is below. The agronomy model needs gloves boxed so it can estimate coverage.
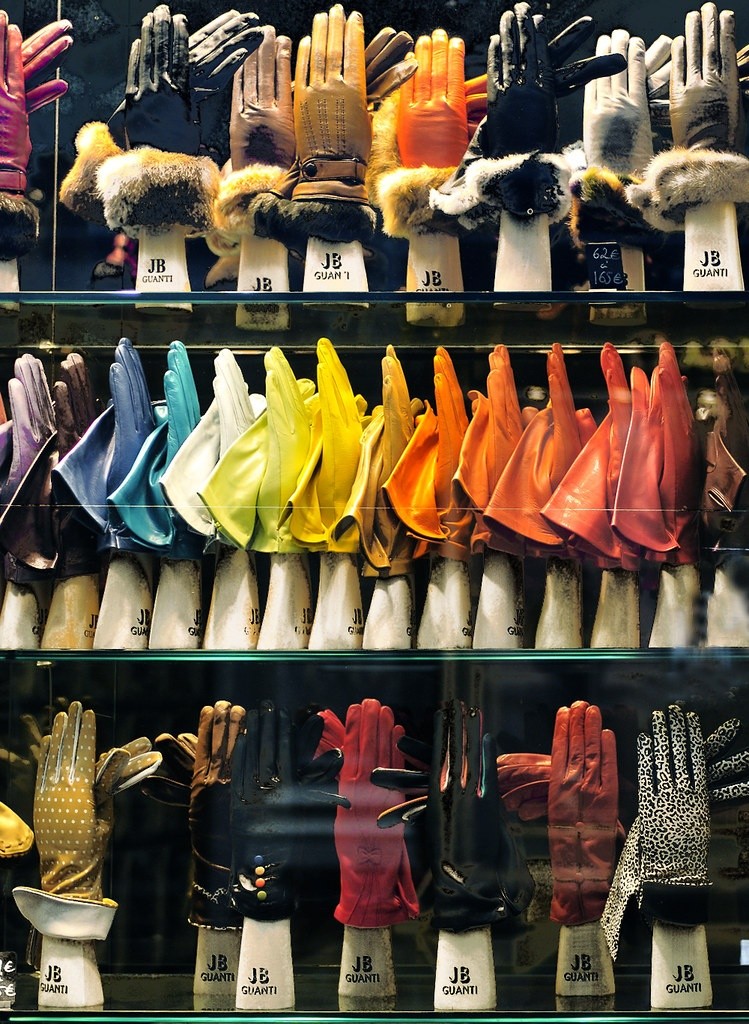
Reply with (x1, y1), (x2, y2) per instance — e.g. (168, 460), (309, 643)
(634, 703), (714, 928)
(497, 753), (626, 866)
(0, 1), (747, 580)
(332, 699), (408, 929)
(229, 700), (309, 922)
(426, 697), (509, 933)
(139, 731), (199, 808)
(546, 700), (618, 927)
(370, 735), (536, 917)
(11, 701), (118, 940)
(599, 717), (749, 962)
(292, 712), (352, 810)
(312, 708), (421, 920)
(93, 736), (162, 808)
(188, 700), (246, 930)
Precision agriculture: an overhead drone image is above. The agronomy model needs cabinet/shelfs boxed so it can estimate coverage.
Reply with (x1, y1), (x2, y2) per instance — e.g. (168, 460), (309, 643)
(0, 290), (749, 1024)
